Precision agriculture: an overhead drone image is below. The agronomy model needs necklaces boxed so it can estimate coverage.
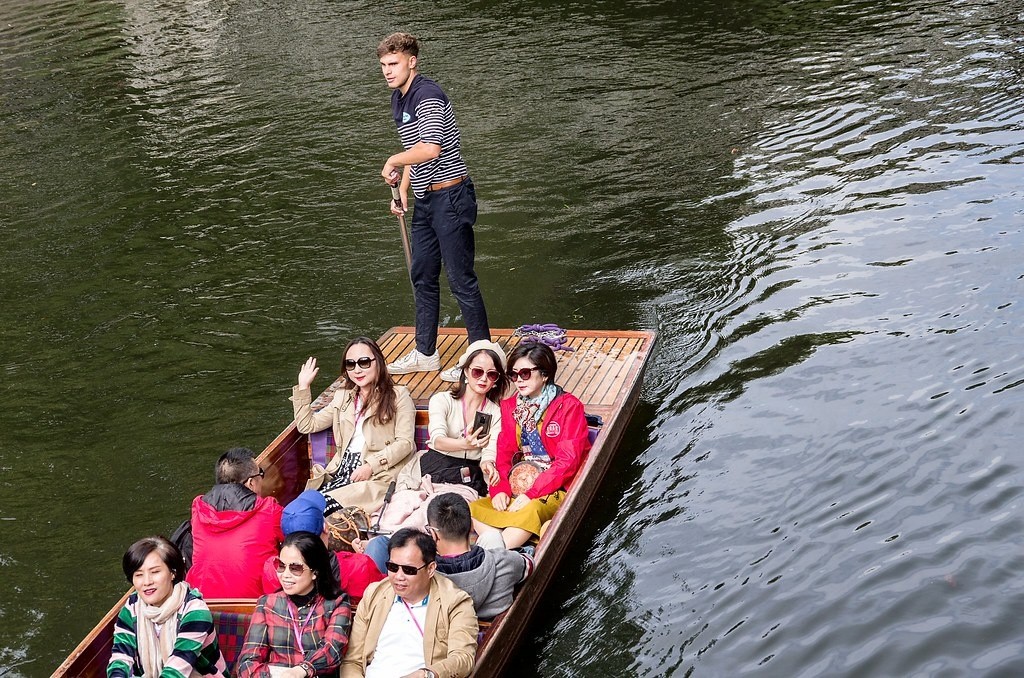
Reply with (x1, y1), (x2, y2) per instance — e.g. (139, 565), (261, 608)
(462, 397), (486, 438)
(354, 390), (370, 428)
(297, 597), (315, 629)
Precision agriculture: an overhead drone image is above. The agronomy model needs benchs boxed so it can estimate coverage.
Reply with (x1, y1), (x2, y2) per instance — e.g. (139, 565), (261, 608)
(204, 599), (490, 678)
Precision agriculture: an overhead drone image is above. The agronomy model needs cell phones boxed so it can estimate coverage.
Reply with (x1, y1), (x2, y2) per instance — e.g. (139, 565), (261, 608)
(471, 411), (492, 439)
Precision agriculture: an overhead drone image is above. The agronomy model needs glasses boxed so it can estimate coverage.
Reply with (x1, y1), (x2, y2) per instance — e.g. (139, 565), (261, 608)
(242, 467), (265, 483)
(385, 558), (435, 575)
(272, 557), (315, 576)
(506, 367), (539, 382)
(344, 357), (375, 371)
(468, 366), (500, 382)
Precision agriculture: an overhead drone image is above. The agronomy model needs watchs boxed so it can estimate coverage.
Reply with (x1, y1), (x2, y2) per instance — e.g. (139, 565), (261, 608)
(419, 668), (437, 678)
(300, 663), (310, 677)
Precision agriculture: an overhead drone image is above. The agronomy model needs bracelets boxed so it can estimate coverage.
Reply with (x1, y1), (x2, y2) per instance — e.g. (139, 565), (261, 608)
(491, 460), (496, 467)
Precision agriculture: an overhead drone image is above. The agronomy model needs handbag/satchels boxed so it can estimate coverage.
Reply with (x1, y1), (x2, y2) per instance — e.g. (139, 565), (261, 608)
(324, 505), (371, 551)
(170, 520), (193, 580)
(508, 450), (545, 498)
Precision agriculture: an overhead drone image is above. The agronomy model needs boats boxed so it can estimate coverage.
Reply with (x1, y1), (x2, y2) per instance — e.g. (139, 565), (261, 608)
(47, 325), (656, 678)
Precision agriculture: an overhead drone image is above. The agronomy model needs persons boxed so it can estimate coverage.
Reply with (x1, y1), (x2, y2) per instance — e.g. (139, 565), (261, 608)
(107, 537), (230, 678)
(261, 489), (389, 608)
(339, 529), (477, 678)
(182, 447), (286, 598)
(426, 492), (534, 617)
(377, 32), (490, 382)
(470, 342), (590, 550)
(392, 340), (507, 538)
(290, 337), (416, 526)
(231, 531), (352, 678)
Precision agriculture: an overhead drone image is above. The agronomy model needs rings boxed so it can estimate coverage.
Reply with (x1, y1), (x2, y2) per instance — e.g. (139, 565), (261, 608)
(477, 443), (481, 447)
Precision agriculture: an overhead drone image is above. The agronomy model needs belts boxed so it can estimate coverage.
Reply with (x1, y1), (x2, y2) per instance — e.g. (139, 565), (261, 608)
(427, 175), (468, 190)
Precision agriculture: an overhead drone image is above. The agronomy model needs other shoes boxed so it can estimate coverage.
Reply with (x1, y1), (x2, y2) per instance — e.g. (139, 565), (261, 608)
(440, 360), (468, 383)
(386, 348), (440, 374)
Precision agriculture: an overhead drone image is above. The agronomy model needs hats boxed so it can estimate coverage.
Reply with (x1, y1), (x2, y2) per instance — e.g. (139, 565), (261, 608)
(459, 339), (507, 372)
(281, 489), (325, 535)
